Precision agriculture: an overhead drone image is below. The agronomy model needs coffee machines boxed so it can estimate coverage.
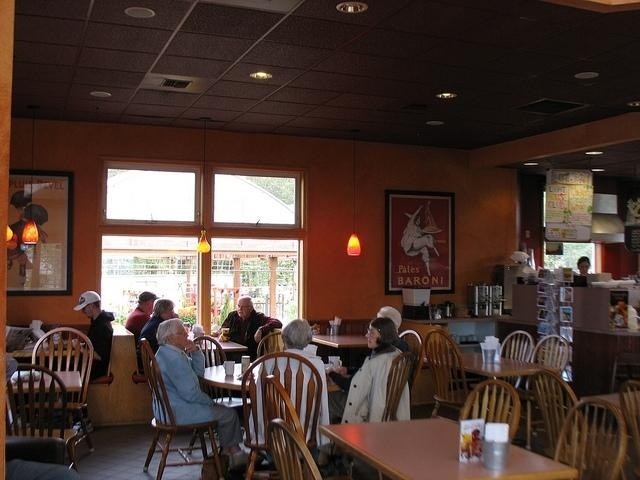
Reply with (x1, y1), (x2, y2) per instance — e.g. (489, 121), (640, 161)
(469, 285), (504, 317)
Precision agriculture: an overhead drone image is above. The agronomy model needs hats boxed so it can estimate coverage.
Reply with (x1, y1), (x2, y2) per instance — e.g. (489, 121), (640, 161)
(73, 290), (101, 311)
(139, 292), (159, 302)
(376, 306), (402, 328)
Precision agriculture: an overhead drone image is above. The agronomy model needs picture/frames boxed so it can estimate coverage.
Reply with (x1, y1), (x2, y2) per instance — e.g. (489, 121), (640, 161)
(5, 168), (75, 297)
(382, 188), (457, 294)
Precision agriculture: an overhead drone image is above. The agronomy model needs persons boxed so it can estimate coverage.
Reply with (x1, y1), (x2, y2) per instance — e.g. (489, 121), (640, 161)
(326, 306), (410, 420)
(8, 190), (49, 259)
(312, 317), (411, 475)
(609, 295), (638, 330)
(57, 291), (115, 434)
(123, 282), (282, 466)
(5, 321), (30, 432)
(249, 318), (331, 466)
(565, 257), (598, 288)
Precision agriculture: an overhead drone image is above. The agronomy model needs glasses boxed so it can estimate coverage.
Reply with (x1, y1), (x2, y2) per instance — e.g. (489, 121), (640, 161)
(367, 330), (378, 338)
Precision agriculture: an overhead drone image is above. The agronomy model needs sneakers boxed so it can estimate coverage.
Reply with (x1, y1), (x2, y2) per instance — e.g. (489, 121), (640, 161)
(229, 450), (264, 466)
(74, 420), (94, 434)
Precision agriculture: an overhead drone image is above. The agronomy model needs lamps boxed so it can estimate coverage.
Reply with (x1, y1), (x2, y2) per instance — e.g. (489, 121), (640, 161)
(192, 118), (217, 254)
(22, 106), (42, 245)
(343, 130), (365, 259)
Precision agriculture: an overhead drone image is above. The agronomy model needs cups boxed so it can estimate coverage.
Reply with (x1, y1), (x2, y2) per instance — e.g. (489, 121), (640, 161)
(483, 440), (508, 470)
(183, 322), (189, 336)
(484, 349), (495, 365)
(223, 361), (234, 376)
(328, 356), (342, 367)
(330, 325), (338, 335)
(193, 333), (204, 345)
(221, 328), (230, 342)
(563, 268), (571, 280)
(241, 355), (250, 374)
(53, 333), (62, 345)
